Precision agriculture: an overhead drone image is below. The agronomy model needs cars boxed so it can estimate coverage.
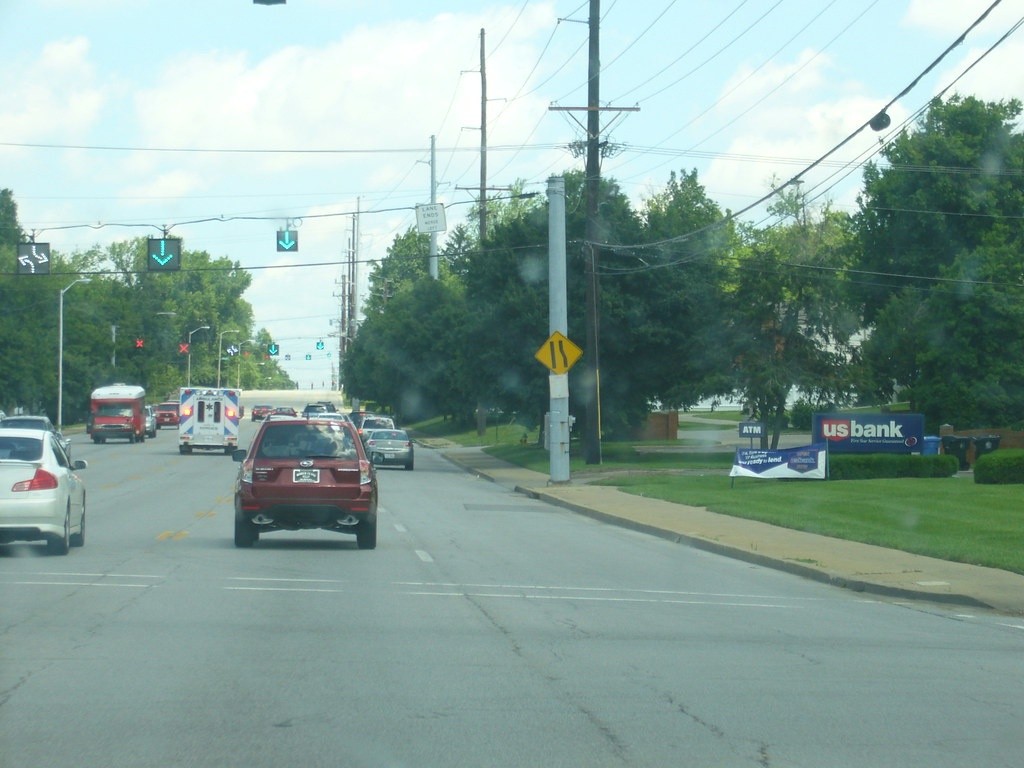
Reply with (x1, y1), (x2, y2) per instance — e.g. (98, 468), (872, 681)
(0, 409), (71, 463)
(251, 401), (397, 443)
(362, 428), (416, 471)
(145, 401), (179, 438)
(0, 431), (89, 555)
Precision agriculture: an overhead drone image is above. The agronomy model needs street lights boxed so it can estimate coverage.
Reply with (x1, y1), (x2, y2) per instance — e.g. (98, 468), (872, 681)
(217, 329), (240, 388)
(57, 277), (93, 432)
(187, 325), (211, 388)
(135, 311), (177, 385)
(237, 339), (256, 389)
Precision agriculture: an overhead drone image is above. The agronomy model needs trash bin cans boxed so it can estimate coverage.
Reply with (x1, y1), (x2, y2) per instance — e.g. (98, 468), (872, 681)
(920, 436), (942, 456)
(975, 435), (999, 459)
(943, 435), (971, 471)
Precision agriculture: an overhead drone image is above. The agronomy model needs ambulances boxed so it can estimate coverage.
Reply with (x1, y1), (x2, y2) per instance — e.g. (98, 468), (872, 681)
(177, 387), (245, 456)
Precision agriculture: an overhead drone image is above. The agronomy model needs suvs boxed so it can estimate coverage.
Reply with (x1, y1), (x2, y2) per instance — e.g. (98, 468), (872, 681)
(231, 412), (386, 550)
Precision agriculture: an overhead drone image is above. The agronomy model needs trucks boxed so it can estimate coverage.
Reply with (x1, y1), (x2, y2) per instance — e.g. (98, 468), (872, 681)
(89, 383), (147, 444)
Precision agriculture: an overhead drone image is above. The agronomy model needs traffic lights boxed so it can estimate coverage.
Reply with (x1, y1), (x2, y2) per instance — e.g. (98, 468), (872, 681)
(306, 355), (312, 360)
